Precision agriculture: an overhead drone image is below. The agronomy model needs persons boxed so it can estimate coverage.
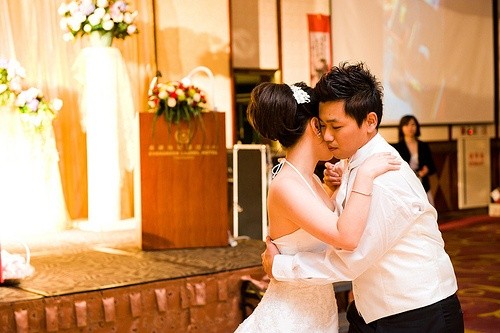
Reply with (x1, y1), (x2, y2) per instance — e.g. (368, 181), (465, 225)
(233, 80), (401, 333)
(395, 115), (438, 207)
(261, 58), (465, 333)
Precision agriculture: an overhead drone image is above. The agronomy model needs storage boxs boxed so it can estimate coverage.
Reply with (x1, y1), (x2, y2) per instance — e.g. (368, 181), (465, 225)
(232, 144), (271, 242)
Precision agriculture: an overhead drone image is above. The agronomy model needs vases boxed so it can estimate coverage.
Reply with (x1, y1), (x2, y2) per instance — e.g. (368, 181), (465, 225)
(89, 31), (114, 47)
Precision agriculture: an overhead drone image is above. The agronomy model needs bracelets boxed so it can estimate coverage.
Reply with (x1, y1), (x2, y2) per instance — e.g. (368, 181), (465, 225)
(351, 189), (373, 197)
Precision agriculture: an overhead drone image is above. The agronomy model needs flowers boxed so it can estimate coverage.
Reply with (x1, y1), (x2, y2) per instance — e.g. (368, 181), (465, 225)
(147, 81), (207, 155)
(0, 58), (63, 130)
(57, 0), (139, 42)
